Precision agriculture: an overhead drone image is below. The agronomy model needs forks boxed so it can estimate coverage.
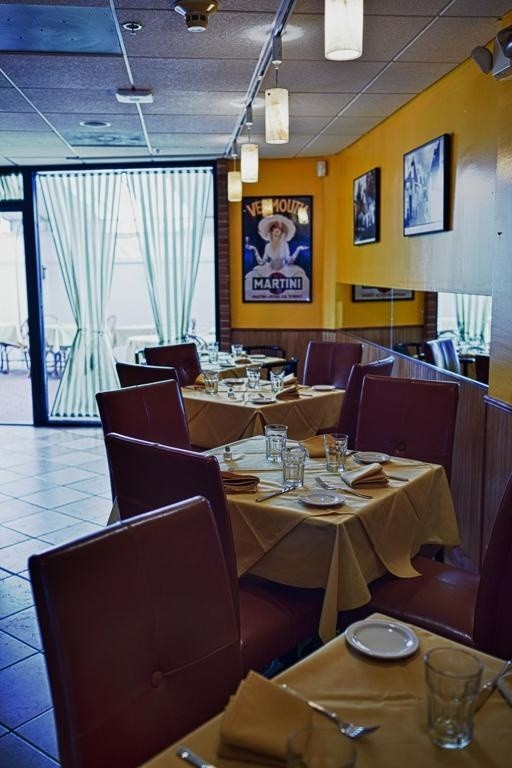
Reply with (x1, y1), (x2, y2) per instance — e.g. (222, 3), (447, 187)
(315, 476), (372, 499)
(279, 680), (380, 741)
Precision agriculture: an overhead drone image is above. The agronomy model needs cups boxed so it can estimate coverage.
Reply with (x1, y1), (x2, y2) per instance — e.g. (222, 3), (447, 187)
(324, 433), (348, 473)
(280, 446), (307, 487)
(201, 342), (287, 404)
(265, 425), (288, 462)
(422, 645), (483, 749)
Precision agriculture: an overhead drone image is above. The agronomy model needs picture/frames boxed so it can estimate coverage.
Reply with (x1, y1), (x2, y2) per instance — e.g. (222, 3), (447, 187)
(351, 167), (381, 246)
(240, 195), (312, 304)
(402, 133), (450, 239)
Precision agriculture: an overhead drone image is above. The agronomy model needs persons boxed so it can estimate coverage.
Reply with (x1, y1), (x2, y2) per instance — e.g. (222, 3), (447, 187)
(244, 214), (310, 300)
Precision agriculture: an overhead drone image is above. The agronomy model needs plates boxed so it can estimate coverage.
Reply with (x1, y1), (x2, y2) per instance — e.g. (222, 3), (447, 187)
(345, 619), (420, 659)
(298, 490), (345, 508)
(352, 452), (391, 464)
(309, 385), (336, 390)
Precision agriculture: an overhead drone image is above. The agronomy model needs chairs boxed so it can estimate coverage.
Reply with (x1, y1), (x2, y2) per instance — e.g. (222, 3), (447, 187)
(1, 314), (196, 378)
(394, 328), (476, 374)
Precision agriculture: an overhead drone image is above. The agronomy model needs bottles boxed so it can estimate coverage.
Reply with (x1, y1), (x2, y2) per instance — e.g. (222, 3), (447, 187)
(224, 445), (232, 462)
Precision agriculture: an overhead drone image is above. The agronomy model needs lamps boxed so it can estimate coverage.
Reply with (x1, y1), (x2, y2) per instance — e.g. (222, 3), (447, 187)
(227, 0), (364, 202)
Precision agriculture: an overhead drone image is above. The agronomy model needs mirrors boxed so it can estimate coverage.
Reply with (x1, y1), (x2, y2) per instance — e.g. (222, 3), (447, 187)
(333, 283), (491, 383)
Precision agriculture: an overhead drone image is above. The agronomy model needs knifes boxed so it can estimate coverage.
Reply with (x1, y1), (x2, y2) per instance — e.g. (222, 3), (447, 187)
(256, 487), (295, 502)
(477, 659), (509, 712)
